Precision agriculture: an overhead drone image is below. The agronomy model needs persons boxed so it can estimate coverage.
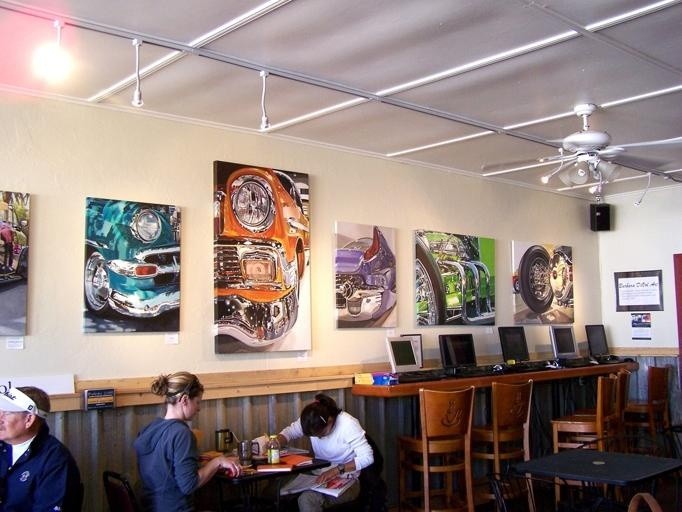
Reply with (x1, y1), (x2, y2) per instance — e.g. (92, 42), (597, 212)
(0, 380), (76, 512)
(267, 393), (374, 512)
(1, 227), (14, 272)
(133, 372), (252, 511)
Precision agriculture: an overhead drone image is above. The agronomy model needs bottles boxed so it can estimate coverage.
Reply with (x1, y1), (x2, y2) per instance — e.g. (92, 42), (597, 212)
(267, 435), (280, 464)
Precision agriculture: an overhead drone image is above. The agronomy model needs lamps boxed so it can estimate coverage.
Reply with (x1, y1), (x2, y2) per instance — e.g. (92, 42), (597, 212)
(541, 148), (563, 184)
(634, 174), (651, 207)
(588, 178), (605, 194)
(558, 156), (621, 187)
(260, 71), (272, 135)
(131, 38), (142, 108)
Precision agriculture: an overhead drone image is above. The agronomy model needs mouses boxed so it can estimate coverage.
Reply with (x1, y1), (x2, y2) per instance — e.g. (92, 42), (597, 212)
(590, 361), (599, 364)
(440, 375), (448, 379)
(624, 358), (634, 362)
(493, 365), (503, 372)
(546, 365), (557, 369)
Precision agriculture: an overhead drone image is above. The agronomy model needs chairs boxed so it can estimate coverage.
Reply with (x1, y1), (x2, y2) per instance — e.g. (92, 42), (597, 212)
(627, 491), (662, 512)
(571, 368), (631, 454)
(103, 470), (139, 512)
(326, 434), (384, 508)
(472, 378), (535, 512)
(396, 386), (476, 512)
(625, 365), (671, 437)
(550, 372), (618, 503)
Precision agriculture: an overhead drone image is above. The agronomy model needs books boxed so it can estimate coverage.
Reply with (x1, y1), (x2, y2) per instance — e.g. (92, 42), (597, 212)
(279, 473), (356, 498)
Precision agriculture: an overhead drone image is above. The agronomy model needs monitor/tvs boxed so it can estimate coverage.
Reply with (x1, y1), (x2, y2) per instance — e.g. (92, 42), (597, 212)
(585, 325), (610, 357)
(439, 334), (477, 375)
(498, 326), (531, 364)
(549, 325), (579, 358)
(385, 336), (419, 373)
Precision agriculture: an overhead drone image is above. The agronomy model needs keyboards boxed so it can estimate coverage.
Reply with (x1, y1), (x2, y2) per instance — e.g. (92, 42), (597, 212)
(398, 374), (441, 383)
(600, 360), (623, 364)
(521, 365), (548, 373)
(567, 362), (594, 368)
(457, 371), (487, 377)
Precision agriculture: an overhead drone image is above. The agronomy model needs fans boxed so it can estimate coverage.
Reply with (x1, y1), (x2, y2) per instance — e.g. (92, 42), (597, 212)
(482, 103), (682, 178)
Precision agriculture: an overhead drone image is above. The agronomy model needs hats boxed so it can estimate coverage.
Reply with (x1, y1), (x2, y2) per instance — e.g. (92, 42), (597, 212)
(0, 387), (49, 419)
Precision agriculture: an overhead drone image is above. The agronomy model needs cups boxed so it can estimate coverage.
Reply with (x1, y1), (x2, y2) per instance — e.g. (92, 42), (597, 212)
(216, 429), (233, 452)
(238, 440), (259, 466)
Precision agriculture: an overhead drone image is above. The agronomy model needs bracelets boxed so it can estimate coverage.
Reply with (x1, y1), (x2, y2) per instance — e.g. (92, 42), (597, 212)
(337, 463), (344, 474)
(210, 460), (223, 469)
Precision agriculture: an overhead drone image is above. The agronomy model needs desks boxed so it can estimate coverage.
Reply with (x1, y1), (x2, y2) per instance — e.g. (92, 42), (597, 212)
(519, 447), (681, 511)
(216, 447), (331, 512)
(353, 362), (640, 511)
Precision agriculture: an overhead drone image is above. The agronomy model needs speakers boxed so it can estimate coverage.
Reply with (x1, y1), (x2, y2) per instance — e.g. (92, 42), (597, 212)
(590, 203), (610, 231)
